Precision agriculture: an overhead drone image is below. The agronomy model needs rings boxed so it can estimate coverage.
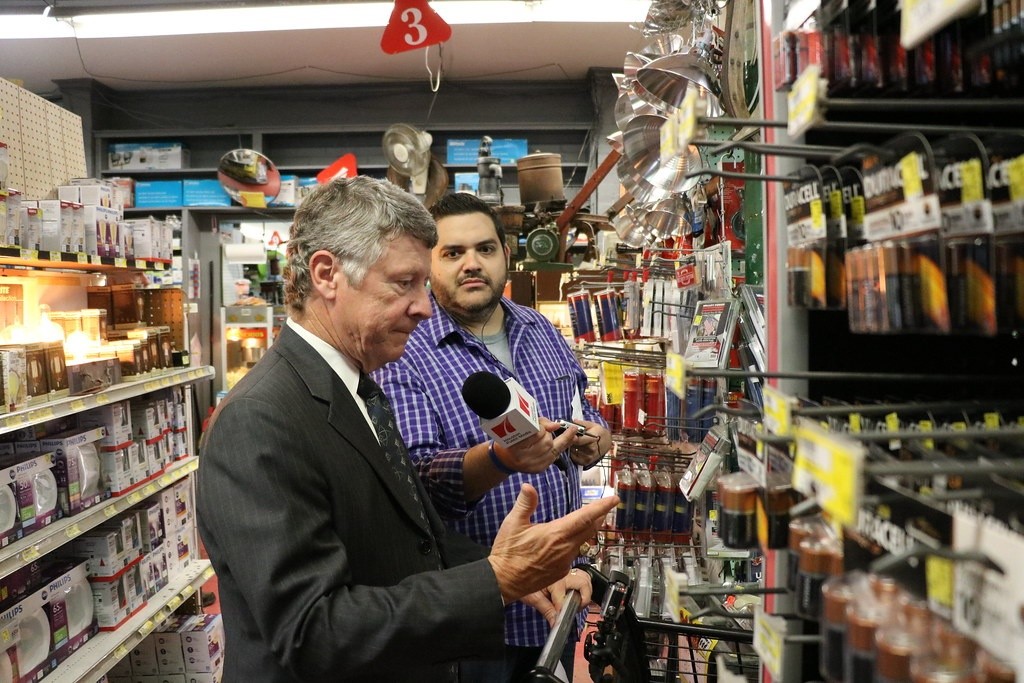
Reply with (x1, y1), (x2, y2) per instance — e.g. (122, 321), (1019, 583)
(580, 541), (590, 557)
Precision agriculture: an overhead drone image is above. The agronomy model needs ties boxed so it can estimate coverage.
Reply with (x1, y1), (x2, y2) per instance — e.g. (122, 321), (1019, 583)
(357, 367), (445, 572)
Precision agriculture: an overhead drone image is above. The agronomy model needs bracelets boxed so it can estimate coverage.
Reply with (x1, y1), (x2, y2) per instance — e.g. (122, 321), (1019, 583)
(488, 439), (517, 474)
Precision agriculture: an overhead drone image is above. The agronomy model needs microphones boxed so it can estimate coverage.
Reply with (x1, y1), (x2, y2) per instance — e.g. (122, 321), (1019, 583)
(461, 371), (568, 472)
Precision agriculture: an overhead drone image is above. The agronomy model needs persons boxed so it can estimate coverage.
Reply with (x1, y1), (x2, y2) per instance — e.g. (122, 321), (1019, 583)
(371, 194), (611, 683)
(194, 174), (620, 683)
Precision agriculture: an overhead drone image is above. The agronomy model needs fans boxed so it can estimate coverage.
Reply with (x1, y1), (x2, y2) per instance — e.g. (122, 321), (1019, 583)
(381, 123), (436, 207)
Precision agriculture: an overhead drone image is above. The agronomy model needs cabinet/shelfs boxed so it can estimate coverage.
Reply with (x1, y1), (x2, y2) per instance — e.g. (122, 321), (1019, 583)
(0, 364), (213, 682)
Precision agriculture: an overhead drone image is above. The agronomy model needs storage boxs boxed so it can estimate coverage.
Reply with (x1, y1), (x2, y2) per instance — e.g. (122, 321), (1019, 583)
(0, 399), (224, 682)
(447, 137), (529, 167)
(17, 141), (323, 264)
